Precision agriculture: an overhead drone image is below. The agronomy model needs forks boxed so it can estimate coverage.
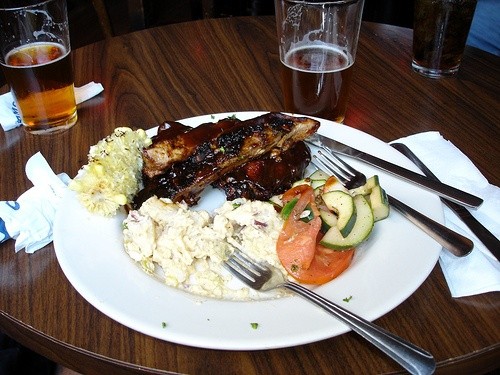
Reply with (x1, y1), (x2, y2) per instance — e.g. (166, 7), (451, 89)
(221, 245), (438, 375)
(307, 144), (474, 258)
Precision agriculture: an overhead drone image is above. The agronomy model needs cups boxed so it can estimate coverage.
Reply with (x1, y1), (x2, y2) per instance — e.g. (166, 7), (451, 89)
(274, 0), (364, 75)
(411, 0), (477, 79)
(1, 0), (79, 137)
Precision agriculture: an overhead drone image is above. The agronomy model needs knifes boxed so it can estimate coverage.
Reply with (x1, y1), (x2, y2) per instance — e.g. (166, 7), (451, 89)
(302, 133), (484, 211)
(389, 143), (499, 264)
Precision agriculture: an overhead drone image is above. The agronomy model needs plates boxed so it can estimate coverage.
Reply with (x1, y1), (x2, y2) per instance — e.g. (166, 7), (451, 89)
(52, 111), (446, 351)
